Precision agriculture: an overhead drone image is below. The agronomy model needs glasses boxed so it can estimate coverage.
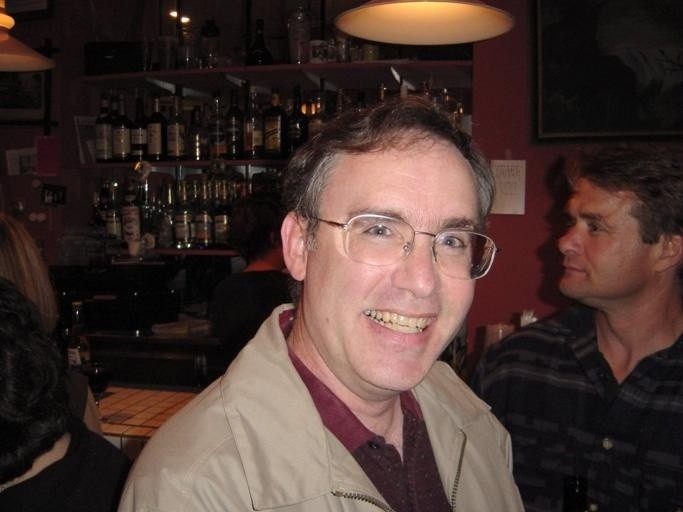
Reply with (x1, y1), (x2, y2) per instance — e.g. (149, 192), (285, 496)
(307, 214), (502, 281)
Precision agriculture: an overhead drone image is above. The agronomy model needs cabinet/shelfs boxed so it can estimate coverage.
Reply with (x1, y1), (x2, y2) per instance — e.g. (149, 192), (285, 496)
(69, 1), (473, 260)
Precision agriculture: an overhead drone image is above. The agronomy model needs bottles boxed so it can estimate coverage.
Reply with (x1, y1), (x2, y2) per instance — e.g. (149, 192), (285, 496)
(336, 35), (350, 62)
(96, 172), (253, 253)
(59, 298), (94, 366)
(136, 3), (310, 73)
(95, 76), (462, 164)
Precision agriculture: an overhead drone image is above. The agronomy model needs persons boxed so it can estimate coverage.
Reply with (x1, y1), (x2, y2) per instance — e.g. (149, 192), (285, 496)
(208, 190), (292, 385)
(0, 277), (132, 512)
(467, 142), (683, 512)
(118, 95), (524, 512)
(0, 212), (101, 437)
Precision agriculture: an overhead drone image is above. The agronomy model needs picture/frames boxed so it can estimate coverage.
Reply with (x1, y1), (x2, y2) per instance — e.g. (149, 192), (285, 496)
(528, 1), (682, 145)
(0, 39), (50, 125)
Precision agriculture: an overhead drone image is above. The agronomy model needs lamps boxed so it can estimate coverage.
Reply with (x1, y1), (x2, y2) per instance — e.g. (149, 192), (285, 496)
(329, 0), (520, 48)
(0, 0), (57, 74)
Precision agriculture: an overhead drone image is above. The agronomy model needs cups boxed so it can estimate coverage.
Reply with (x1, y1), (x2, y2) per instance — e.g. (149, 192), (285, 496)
(362, 43), (379, 60)
(483, 324), (514, 350)
(307, 38), (336, 62)
